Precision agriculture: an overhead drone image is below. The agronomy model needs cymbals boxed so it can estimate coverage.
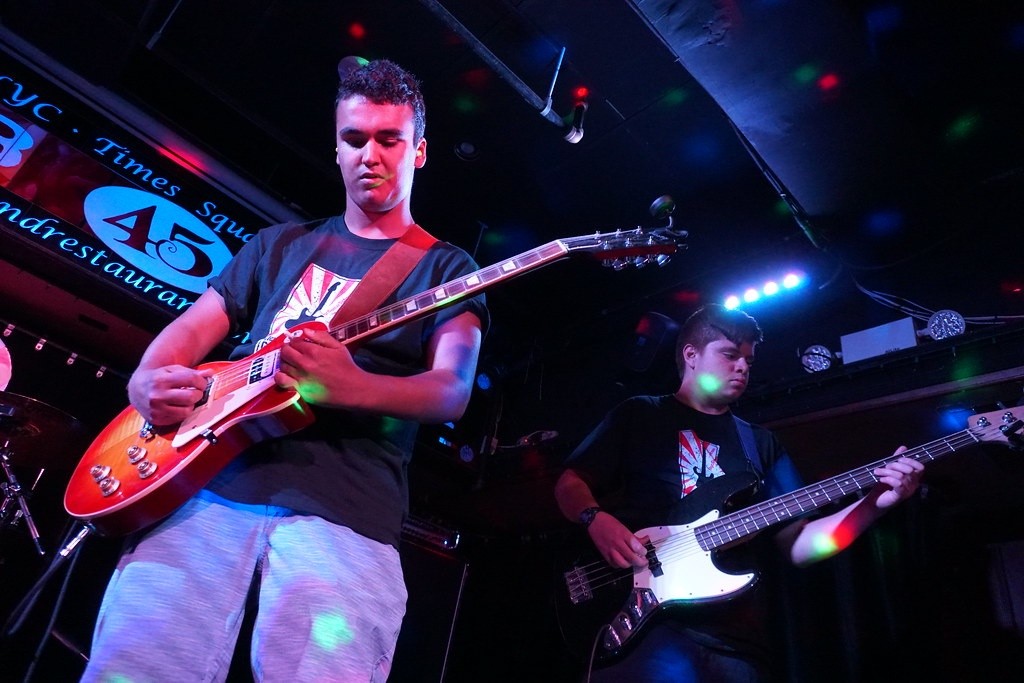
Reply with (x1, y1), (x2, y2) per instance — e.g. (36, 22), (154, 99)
(0, 390), (81, 447)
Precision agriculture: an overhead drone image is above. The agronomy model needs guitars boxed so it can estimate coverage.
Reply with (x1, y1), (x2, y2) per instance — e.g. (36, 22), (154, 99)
(64, 194), (691, 537)
(556, 399), (1024, 662)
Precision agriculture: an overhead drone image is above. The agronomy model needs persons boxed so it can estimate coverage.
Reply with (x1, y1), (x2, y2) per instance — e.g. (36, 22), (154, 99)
(81, 60), (488, 683)
(555, 301), (924, 683)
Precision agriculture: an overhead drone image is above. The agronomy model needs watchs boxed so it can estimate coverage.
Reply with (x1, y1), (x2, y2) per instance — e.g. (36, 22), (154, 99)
(578, 507), (603, 533)
